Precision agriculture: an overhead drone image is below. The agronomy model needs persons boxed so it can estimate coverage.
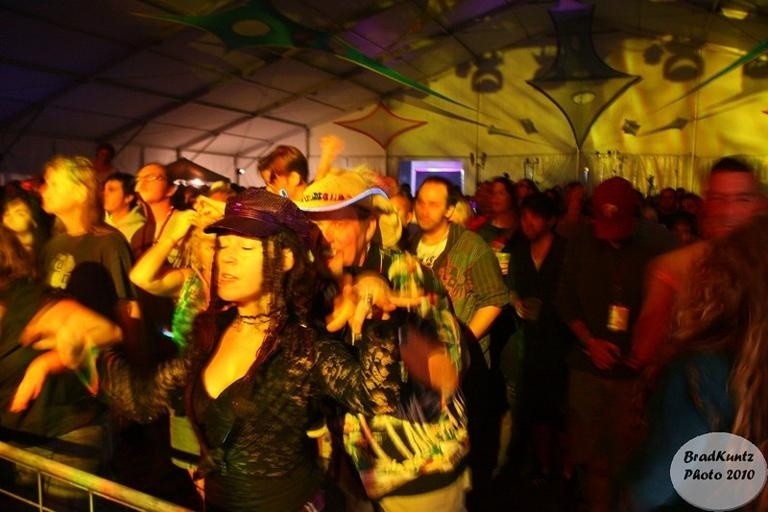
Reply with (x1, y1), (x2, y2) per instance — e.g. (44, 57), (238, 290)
(0, 145), (765, 512)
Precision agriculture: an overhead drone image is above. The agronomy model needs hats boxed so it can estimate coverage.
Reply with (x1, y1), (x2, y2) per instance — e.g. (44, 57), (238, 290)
(292, 167), (402, 250)
(204, 189), (311, 252)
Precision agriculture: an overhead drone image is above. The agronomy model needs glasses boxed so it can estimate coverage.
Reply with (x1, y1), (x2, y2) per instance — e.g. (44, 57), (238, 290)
(133, 174), (168, 184)
